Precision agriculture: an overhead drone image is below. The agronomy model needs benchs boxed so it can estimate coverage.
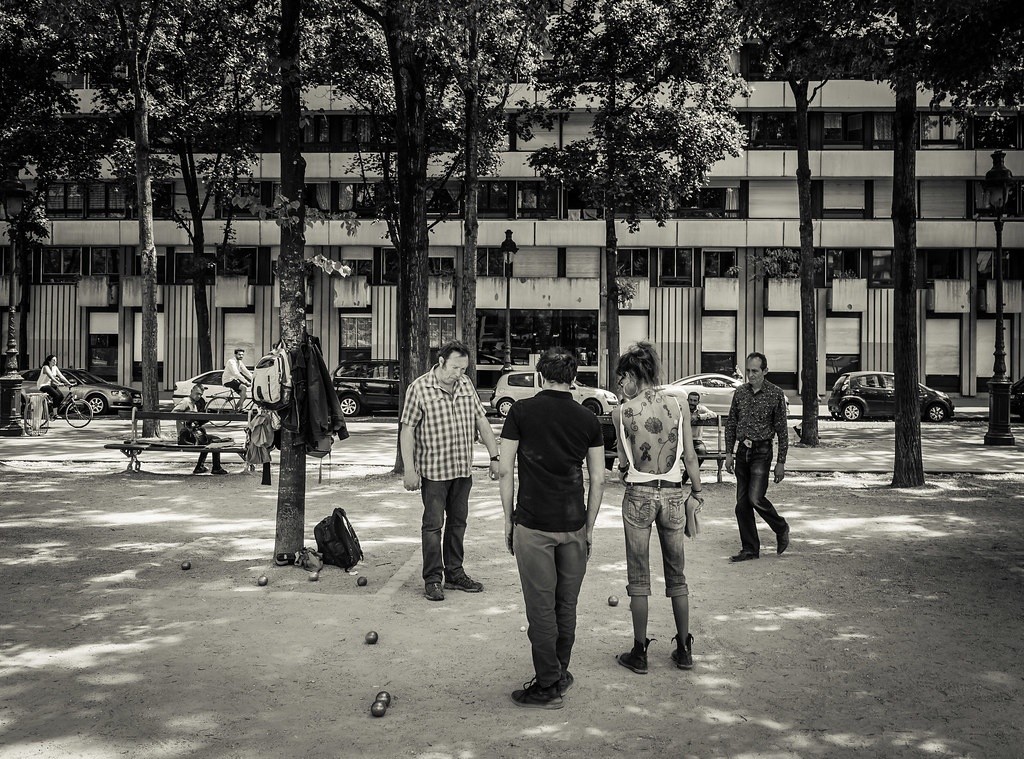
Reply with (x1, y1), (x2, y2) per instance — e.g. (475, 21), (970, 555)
(105, 410), (262, 472)
(600, 414), (736, 482)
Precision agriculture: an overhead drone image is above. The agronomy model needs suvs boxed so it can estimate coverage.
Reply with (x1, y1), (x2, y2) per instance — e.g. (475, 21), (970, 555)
(15, 368), (145, 415)
(488, 369), (619, 417)
(328, 358), (401, 417)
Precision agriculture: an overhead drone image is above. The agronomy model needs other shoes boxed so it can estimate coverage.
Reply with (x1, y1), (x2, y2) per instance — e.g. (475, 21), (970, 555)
(193, 465), (208, 473)
(52, 414), (62, 419)
(775, 525), (789, 555)
(210, 469), (229, 474)
(730, 549), (760, 561)
(235, 404), (245, 413)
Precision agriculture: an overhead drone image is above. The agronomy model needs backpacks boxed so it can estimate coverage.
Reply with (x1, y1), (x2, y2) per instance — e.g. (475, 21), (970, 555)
(252, 338), (294, 411)
(304, 414), (331, 457)
(313, 508), (364, 573)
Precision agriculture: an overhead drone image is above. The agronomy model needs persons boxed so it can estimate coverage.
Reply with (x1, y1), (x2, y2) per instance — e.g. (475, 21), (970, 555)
(725, 352), (789, 562)
(680, 392), (718, 484)
(400, 340), (500, 600)
(222, 348), (253, 415)
(497, 347), (606, 709)
(612, 339), (704, 675)
(171, 384), (228, 475)
(36, 355), (75, 420)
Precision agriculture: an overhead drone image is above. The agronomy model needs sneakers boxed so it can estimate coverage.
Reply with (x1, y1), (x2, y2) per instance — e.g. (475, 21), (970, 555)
(557, 671), (574, 697)
(425, 582), (444, 600)
(444, 574), (484, 592)
(510, 675), (563, 709)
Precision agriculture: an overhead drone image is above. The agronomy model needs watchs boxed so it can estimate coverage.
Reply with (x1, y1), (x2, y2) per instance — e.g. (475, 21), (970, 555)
(489, 454), (502, 462)
(696, 414), (701, 420)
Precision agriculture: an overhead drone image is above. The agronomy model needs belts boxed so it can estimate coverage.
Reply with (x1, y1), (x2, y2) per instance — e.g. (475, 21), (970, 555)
(626, 479), (682, 488)
(738, 439), (771, 449)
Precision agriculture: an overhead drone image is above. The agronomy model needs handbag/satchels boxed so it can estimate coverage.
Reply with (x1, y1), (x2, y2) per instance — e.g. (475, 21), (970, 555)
(177, 426), (209, 446)
(293, 547), (323, 572)
(259, 408), (282, 431)
(245, 442), (271, 464)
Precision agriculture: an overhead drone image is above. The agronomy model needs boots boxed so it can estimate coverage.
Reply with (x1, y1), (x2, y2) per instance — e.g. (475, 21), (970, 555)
(670, 632), (694, 669)
(619, 637), (657, 674)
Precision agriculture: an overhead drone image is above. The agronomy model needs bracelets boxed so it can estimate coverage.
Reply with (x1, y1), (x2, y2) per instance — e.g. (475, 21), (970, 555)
(618, 463), (628, 472)
(690, 486), (703, 494)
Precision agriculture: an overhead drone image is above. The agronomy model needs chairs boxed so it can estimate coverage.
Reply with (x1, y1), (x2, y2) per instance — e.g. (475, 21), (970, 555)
(307, 437), (332, 487)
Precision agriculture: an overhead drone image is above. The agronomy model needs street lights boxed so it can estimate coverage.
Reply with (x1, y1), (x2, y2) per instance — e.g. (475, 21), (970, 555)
(499, 226), (521, 373)
(0, 166), (33, 437)
(982, 149), (1016, 446)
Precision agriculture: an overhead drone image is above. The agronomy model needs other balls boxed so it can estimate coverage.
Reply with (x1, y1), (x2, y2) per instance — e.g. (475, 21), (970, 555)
(182, 561), (191, 570)
(521, 627), (526, 632)
(309, 572), (319, 581)
(358, 577), (367, 587)
(608, 595), (619, 607)
(366, 631), (378, 644)
(258, 576), (268, 586)
(371, 691), (390, 717)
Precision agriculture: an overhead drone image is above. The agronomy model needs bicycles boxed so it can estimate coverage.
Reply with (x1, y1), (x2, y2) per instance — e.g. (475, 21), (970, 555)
(205, 386), (258, 427)
(22, 380), (95, 427)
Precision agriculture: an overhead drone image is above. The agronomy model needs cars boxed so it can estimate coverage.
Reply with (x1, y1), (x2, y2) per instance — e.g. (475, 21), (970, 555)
(171, 369), (259, 410)
(1010, 376), (1024, 419)
(652, 373), (791, 414)
(827, 371), (955, 423)
